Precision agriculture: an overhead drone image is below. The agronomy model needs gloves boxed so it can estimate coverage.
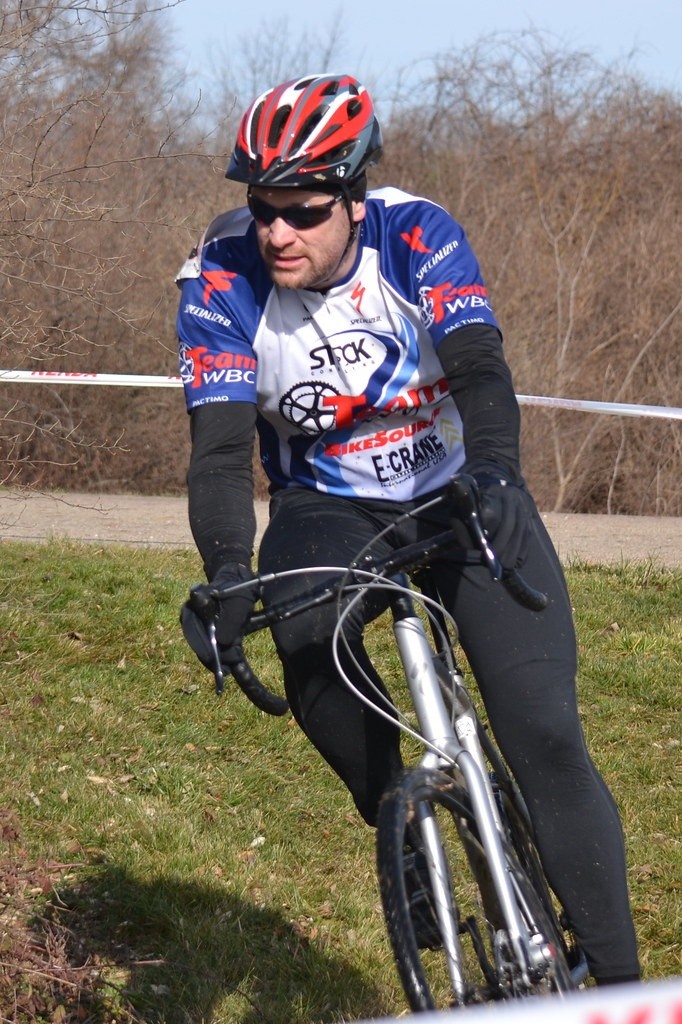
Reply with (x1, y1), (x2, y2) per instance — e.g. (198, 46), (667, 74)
(453, 483), (538, 572)
(183, 564), (257, 678)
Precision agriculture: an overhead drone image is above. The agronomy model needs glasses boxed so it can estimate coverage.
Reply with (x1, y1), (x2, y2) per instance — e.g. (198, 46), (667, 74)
(248, 183), (347, 227)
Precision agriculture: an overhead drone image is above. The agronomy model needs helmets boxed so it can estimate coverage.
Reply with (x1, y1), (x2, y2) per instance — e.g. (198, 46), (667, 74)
(225, 74), (383, 189)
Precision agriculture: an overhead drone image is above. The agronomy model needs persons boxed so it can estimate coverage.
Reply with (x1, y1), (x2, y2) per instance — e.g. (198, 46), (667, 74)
(174, 76), (641, 995)
